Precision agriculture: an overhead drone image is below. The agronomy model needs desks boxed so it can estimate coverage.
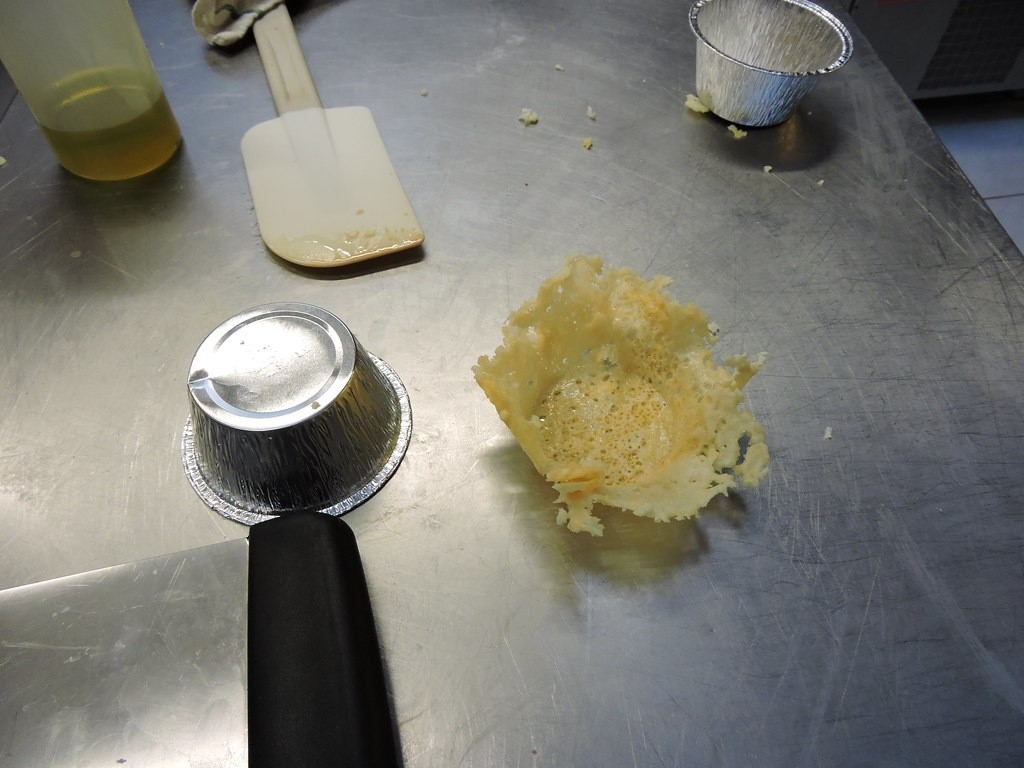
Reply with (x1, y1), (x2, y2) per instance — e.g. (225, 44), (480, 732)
(0, 0), (1024, 768)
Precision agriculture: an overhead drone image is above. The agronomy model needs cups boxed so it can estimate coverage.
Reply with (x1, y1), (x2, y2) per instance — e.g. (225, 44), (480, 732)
(0, 0), (182, 181)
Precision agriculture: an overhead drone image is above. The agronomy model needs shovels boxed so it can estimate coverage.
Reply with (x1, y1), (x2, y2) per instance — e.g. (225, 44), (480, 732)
(239, 0), (426, 270)
